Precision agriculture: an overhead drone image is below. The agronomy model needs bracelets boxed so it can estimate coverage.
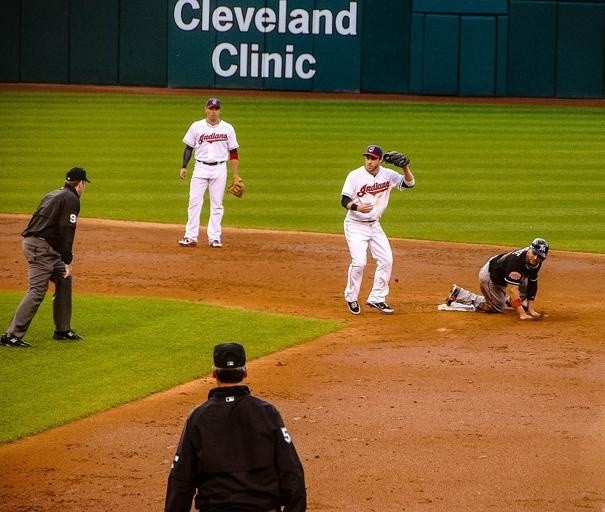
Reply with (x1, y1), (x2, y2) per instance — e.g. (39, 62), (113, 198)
(350, 204), (359, 210)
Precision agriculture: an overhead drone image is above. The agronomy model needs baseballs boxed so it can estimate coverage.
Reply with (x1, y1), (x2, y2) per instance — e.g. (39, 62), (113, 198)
(385, 156), (391, 160)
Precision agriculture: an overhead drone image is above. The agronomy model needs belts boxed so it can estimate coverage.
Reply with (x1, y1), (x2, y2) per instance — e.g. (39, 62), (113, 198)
(197, 161), (224, 166)
(353, 218), (375, 223)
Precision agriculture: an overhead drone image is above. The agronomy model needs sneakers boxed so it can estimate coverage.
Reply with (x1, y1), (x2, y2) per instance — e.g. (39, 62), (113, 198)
(366, 300), (394, 313)
(178, 238), (197, 246)
(53, 328), (83, 340)
(445, 284), (461, 306)
(0, 333), (32, 348)
(345, 300), (360, 314)
(211, 240), (222, 247)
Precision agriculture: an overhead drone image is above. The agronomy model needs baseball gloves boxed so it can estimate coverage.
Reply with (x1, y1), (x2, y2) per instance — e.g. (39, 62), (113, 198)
(383, 151), (408, 167)
(226, 183), (243, 197)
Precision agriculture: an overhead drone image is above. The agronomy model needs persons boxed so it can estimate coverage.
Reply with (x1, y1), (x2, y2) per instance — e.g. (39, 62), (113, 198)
(446, 236), (548, 320)
(1, 165), (85, 347)
(166, 342), (308, 511)
(178, 96), (244, 247)
(339, 145), (416, 314)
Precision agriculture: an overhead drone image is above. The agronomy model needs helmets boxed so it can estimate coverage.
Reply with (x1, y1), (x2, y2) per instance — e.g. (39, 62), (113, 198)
(532, 237), (549, 259)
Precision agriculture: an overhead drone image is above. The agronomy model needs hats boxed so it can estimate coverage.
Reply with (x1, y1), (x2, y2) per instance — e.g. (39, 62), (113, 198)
(206, 98), (222, 109)
(213, 343), (246, 369)
(66, 168), (90, 183)
(362, 145), (382, 159)
